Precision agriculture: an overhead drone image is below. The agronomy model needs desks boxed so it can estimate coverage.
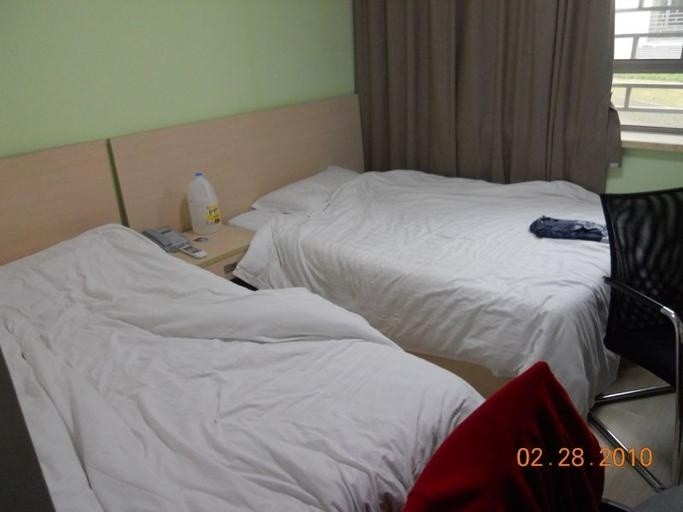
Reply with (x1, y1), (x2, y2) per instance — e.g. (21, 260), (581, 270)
(158, 224), (255, 283)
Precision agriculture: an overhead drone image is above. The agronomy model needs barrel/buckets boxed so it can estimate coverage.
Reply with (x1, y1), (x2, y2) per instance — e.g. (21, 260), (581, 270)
(185, 172), (222, 236)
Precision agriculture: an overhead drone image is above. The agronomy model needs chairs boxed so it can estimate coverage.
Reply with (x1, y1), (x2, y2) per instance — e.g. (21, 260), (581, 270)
(589, 185), (683, 493)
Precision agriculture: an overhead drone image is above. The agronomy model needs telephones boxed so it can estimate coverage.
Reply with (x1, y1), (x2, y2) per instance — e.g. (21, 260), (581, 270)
(141, 226), (189, 252)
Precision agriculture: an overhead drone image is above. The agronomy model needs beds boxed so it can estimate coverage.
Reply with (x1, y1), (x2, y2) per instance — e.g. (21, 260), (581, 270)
(109, 92), (624, 418)
(1, 137), (487, 511)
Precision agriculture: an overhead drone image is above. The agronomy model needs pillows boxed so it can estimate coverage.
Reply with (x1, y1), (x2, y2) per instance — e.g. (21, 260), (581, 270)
(252, 164), (361, 214)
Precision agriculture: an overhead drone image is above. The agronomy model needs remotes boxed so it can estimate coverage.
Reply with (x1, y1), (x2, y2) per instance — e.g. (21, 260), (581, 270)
(181, 245), (207, 259)
